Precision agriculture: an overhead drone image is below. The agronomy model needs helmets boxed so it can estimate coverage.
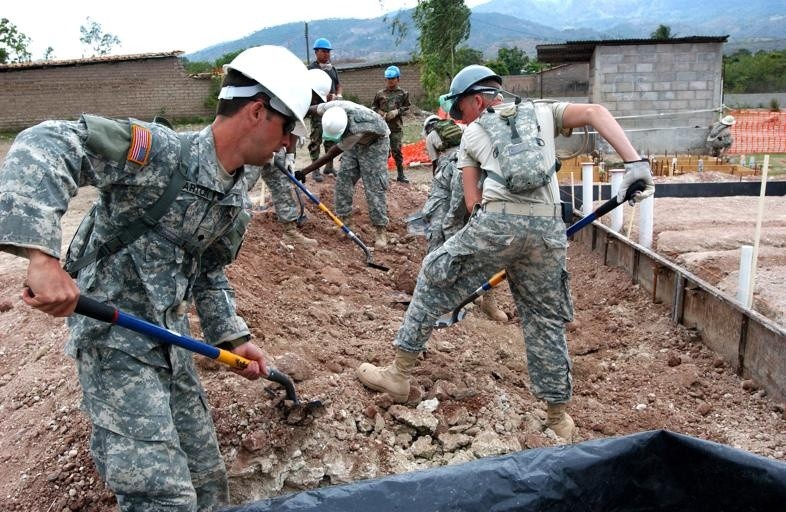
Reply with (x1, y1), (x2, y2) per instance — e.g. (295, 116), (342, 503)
(449, 65), (502, 120)
(222, 38), (348, 142)
(384, 66), (400, 79)
(423, 115), (442, 136)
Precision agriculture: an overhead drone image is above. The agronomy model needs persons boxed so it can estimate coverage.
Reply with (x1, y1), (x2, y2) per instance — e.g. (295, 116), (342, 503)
(706, 115), (736, 157)
(357, 63), (656, 442)
(242, 69), (332, 245)
(424, 147), (510, 323)
(295, 99), (391, 251)
(306, 37), (344, 182)
(423, 114), (465, 179)
(371, 65), (411, 183)
(0, 44), (313, 512)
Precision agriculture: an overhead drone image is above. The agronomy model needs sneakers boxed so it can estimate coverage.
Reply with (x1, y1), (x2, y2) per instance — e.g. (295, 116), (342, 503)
(323, 167), (338, 176)
(312, 169), (323, 182)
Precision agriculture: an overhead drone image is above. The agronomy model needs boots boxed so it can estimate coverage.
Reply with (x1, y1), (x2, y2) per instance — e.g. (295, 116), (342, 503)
(375, 226), (387, 250)
(397, 166), (410, 182)
(357, 348), (418, 403)
(283, 222), (316, 244)
(482, 288), (508, 322)
(546, 401), (575, 439)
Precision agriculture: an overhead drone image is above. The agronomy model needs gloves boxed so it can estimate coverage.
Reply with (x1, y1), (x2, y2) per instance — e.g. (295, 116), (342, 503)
(298, 136), (306, 149)
(385, 110), (398, 122)
(285, 153), (295, 177)
(617, 160), (655, 207)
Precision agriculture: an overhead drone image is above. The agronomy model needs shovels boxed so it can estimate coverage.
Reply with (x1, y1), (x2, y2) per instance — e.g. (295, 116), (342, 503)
(273, 159), (390, 272)
(28, 287), (322, 417)
(432, 179), (647, 329)
(287, 165), (311, 229)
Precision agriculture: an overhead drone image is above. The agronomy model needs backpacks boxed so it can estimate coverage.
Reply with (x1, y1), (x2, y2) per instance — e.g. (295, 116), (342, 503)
(475, 102), (547, 194)
(435, 119), (463, 146)
